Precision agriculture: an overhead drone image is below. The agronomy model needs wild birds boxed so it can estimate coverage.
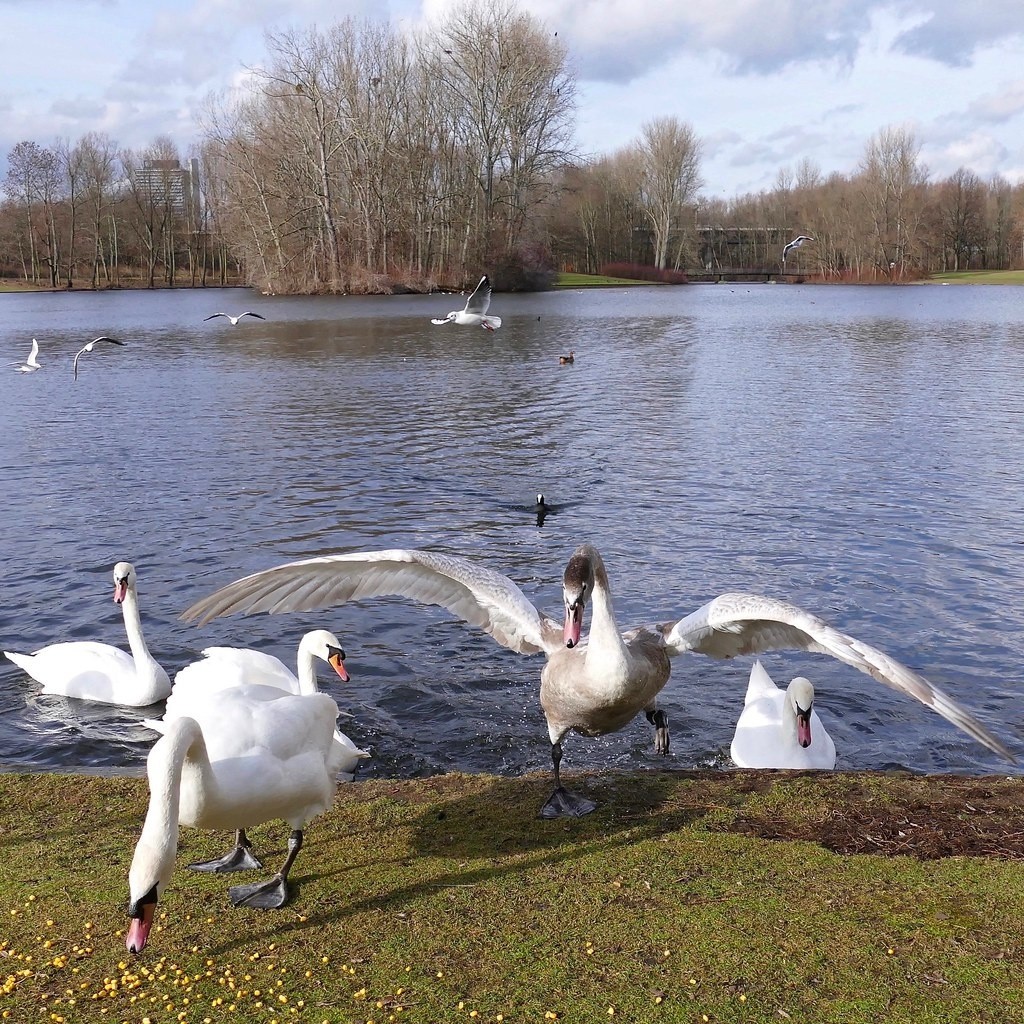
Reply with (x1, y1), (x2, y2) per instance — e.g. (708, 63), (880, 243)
(202, 310), (267, 327)
(13, 339), (42, 374)
(782, 235), (813, 263)
(431, 274), (502, 333)
(74, 336), (126, 382)
(535, 494), (547, 508)
(559, 350), (576, 364)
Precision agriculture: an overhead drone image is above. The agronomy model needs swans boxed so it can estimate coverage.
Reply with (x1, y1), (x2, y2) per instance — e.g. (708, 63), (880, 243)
(122, 627), (370, 954)
(179, 540), (1023, 820)
(3, 561), (170, 709)
